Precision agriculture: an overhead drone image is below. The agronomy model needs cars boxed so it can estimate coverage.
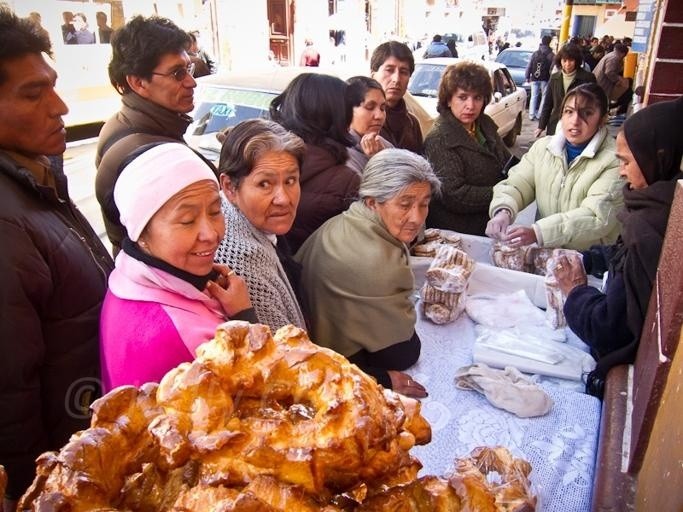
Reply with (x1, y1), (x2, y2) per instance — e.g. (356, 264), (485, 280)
(401, 51), (531, 163)
(64, 63), (439, 304)
(493, 48), (546, 110)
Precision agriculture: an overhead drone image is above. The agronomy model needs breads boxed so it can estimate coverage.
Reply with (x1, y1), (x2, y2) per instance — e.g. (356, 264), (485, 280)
(16, 321), (537, 512)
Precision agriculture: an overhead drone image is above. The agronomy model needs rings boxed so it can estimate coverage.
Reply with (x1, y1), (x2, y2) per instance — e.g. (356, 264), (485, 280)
(408, 379), (412, 387)
(557, 263), (562, 269)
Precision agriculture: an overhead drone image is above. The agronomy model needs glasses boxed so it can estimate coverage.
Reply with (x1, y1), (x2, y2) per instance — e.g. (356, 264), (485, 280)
(148, 63), (197, 81)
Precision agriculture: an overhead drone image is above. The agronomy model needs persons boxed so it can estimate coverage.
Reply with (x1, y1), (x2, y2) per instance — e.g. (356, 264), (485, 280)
(422, 35), (458, 59)
(98, 142), (258, 396)
(552, 101), (682, 359)
(0, 10), (115, 499)
(523, 34), (632, 137)
(31, 11), (113, 46)
(186, 27), (219, 78)
(214, 115), (428, 398)
(94, 16), (220, 257)
(425, 64), (522, 235)
(485, 84), (629, 251)
(288, 147), (441, 368)
(498, 42), (522, 54)
(268, 34), (421, 260)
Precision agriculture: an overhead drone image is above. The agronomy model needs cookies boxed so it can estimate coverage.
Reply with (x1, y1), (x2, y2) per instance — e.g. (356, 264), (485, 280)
(412, 228), (470, 325)
(490, 240), (567, 329)
